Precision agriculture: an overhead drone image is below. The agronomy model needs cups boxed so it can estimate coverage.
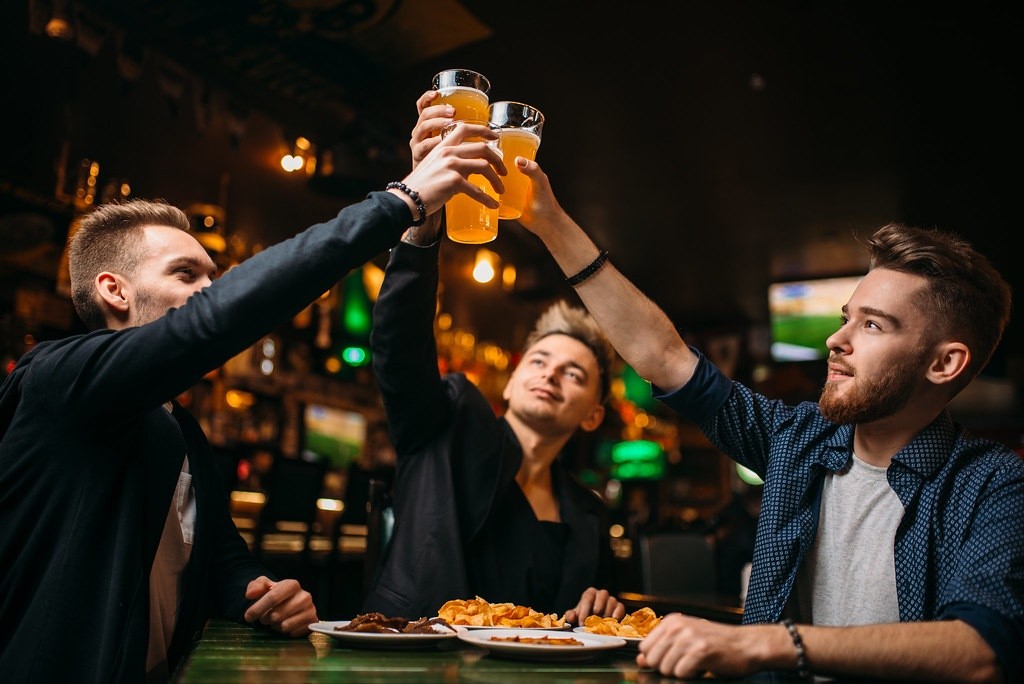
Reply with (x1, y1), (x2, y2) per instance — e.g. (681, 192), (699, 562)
(440, 119), (503, 244)
(431, 69), (490, 138)
(488, 100), (545, 220)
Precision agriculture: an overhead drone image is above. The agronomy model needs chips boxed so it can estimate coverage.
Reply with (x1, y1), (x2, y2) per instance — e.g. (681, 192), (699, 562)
(437, 594), (566, 628)
(583, 606), (664, 638)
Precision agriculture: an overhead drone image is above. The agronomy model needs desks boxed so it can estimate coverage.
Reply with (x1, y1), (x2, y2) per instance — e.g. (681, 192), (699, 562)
(179, 620), (812, 684)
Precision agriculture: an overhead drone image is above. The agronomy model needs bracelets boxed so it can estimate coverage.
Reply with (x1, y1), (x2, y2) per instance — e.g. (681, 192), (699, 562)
(566, 250), (608, 287)
(780, 617), (812, 684)
(383, 181), (426, 226)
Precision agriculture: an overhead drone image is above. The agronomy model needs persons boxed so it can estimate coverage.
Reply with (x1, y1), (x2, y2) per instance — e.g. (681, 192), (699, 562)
(677, 297), (749, 379)
(0, 125), (509, 684)
(369, 89), (625, 626)
(514, 155), (1024, 684)
(332, 404), (398, 543)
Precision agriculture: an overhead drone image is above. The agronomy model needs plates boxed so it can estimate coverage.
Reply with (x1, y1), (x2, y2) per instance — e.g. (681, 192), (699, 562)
(308, 621), (468, 649)
(456, 629), (627, 661)
(573, 627), (645, 645)
(429, 617), (571, 631)
